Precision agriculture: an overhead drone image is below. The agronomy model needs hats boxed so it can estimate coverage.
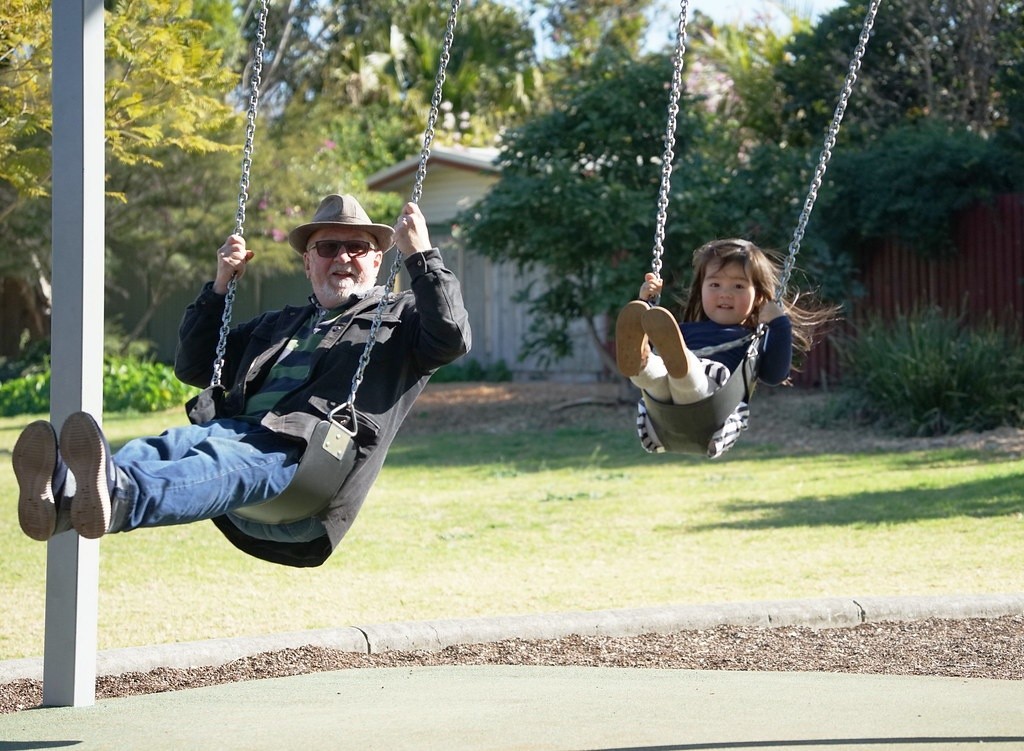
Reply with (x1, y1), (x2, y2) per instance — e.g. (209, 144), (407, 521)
(288, 194), (395, 255)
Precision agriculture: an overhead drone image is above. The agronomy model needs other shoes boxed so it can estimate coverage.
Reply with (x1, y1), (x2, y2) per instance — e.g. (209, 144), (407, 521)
(59, 411), (117, 539)
(640, 307), (688, 379)
(12, 419), (68, 541)
(615, 299), (650, 377)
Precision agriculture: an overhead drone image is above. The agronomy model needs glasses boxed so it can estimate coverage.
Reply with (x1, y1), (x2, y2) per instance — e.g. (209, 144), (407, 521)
(307, 240), (378, 259)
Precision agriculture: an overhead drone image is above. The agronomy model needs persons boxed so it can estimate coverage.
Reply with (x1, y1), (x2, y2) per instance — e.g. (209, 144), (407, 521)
(12, 194), (472, 567)
(616, 238), (833, 466)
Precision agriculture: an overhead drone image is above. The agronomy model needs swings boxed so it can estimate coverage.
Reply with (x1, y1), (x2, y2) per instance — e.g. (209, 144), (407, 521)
(640, 0), (881, 441)
(193, 0), (466, 528)
